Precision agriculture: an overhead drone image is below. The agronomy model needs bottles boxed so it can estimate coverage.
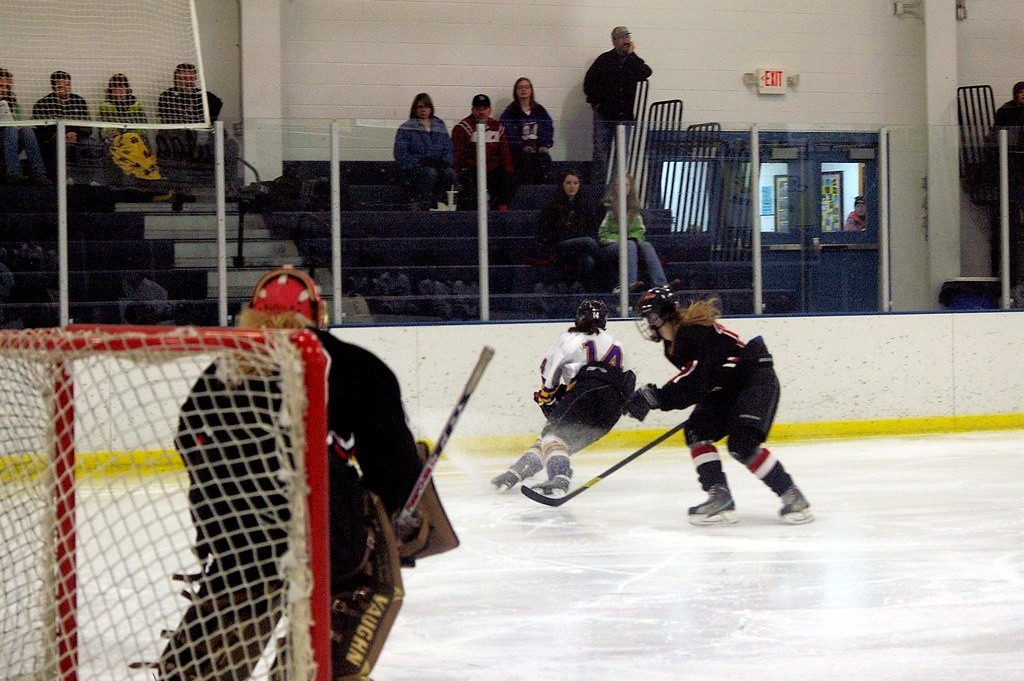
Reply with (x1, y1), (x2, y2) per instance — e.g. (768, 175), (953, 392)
(486, 189), (489, 211)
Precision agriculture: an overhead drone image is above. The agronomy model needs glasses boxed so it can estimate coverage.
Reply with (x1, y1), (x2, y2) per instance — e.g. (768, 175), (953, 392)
(516, 85), (530, 90)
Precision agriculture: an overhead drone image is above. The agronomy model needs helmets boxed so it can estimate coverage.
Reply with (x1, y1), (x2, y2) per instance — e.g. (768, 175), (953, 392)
(636, 287), (680, 343)
(574, 299), (610, 330)
(249, 268), (325, 328)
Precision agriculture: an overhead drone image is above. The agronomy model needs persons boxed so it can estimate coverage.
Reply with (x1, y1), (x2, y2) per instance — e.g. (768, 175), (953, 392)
(844, 195), (866, 231)
(452, 94), (514, 211)
(983, 82), (1024, 308)
(499, 77), (554, 184)
(0, 68), (53, 187)
(156, 64), (239, 186)
(488, 298), (624, 498)
(156, 268), (423, 681)
(597, 172), (674, 292)
(32, 71), (93, 184)
(622, 286), (815, 525)
(583, 27), (652, 183)
(393, 94), (457, 210)
(537, 170), (619, 293)
(96, 73), (168, 188)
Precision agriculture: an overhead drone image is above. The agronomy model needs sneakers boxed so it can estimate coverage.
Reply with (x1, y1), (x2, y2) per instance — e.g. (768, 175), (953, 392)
(531, 469), (572, 499)
(778, 476), (815, 524)
(687, 475), (739, 525)
(490, 464), (534, 493)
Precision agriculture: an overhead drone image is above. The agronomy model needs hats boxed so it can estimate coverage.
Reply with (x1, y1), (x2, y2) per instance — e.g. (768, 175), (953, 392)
(612, 27), (630, 39)
(472, 94), (490, 107)
(855, 196), (866, 206)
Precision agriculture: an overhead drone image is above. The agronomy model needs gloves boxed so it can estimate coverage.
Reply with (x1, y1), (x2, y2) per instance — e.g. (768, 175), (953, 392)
(622, 383), (661, 422)
(391, 507), (434, 558)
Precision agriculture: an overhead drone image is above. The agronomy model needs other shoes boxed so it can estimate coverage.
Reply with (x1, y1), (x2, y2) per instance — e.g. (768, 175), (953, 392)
(668, 277), (684, 290)
(612, 286), (620, 293)
(627, 281), (643, 293)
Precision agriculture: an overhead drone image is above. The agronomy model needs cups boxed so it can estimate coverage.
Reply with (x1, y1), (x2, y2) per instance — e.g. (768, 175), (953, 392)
(446, 191), (458, 211)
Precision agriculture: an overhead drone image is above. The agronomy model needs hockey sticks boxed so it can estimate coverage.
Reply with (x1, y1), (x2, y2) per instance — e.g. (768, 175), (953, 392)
(395, 345), (497, 544)
(521, 418), (688, 508)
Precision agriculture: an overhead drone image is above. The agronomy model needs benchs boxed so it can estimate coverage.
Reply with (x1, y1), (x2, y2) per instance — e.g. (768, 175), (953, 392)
(265, 158), (802, 325)
(0, 145), (243, 328)
